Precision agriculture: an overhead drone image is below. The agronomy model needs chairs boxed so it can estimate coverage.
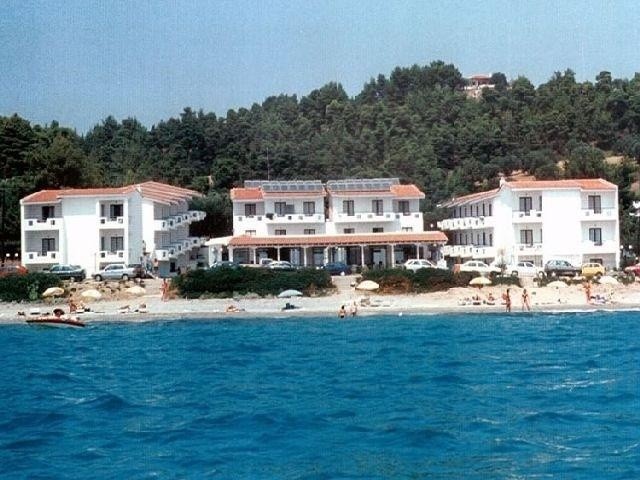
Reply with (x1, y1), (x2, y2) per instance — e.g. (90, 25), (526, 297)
(75, 307), (84, 314)
(120, 305), (147, 314)
(30, 307), (40, 315)
(358, 296), (392, 307)
(458, 299), (506, 305)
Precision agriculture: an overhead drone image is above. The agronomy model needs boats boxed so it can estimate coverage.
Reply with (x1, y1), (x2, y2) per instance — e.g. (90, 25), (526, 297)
(26, 318), (84, 329)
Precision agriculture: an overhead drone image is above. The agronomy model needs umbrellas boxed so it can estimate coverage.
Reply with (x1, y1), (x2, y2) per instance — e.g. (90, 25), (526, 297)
(125, 285), (146, 294)
(42, 286), (65, 300)
(81, 289), (102, 299)
(276, 289), (304, 304)
(355, 280), (380, 292)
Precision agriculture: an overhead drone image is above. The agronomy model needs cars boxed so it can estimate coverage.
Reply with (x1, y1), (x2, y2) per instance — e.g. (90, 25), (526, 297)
(404, 258), (605, 278)
(202, 261), (352, 276)
(0, 264), (29, 278)
(91, 263), (147, 281)
(623, 263), (640, 277)
(37, 264), (84, 282)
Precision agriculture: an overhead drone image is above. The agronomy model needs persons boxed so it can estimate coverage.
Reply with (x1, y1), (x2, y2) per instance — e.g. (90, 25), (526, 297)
(119, 303), (147, 314)
(159, 278), (168, 300)
(337, 305), (347, 320)
(504, 289), (513, 313)
(469, 276), (492, 289)
(521, 289), (531, 312)
(583, 279), (591, 300)
(54, 299), (92, 323)
(140, 239), (159, 276)
(470, 292), (506, 304)
(350, 301), (358, 318)
